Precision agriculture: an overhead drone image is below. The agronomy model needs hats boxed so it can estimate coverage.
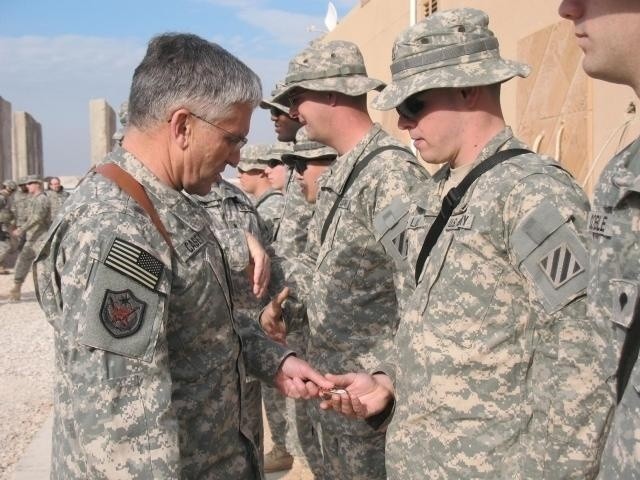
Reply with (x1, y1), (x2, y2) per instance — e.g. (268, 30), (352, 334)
(371, 6), (532, 112)
(259, 39), (387, 115)
(3, 175), (41, 190)
(239, 125), (339, 174)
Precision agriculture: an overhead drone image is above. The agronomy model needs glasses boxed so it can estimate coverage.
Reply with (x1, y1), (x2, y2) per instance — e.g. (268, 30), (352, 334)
(397, 95), (427, 119)
(191, 110), (247, 153)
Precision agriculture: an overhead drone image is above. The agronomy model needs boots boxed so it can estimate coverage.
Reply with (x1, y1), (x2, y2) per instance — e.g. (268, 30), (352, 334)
(262, 445), (293, 471)
(1, 281), (21, 301)
